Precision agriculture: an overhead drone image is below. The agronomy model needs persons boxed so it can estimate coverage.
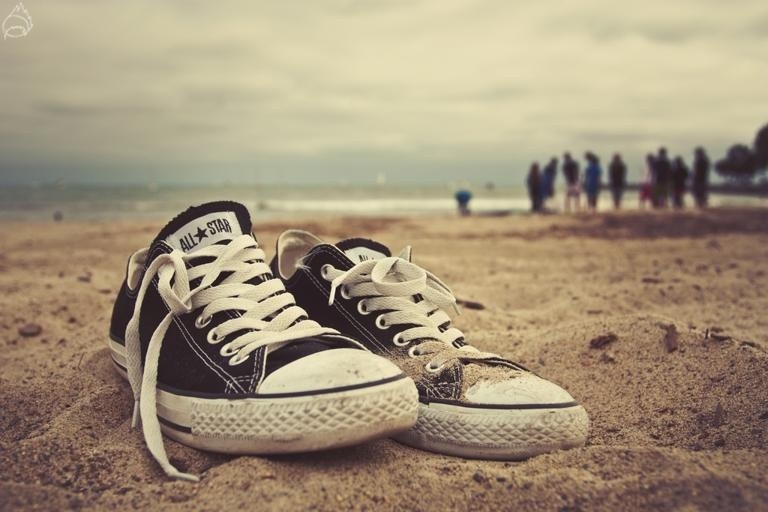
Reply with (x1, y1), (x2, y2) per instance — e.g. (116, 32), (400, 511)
(540, 157), (559, 214)
(670, 154), (691, 209)
(583, 150), (605, 216)
(691, 145), (713, 207)
(526, 162), (545, 214)
(644, 147), (669, 208)
(649, 146), (672, 208)
(607, 152), (628, 211)
(560, 153), (583, 215)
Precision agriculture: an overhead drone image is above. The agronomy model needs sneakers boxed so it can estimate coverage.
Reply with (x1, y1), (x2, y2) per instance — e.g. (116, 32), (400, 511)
(108, 202), (418, 483)
(268, 229), (589, 463)
